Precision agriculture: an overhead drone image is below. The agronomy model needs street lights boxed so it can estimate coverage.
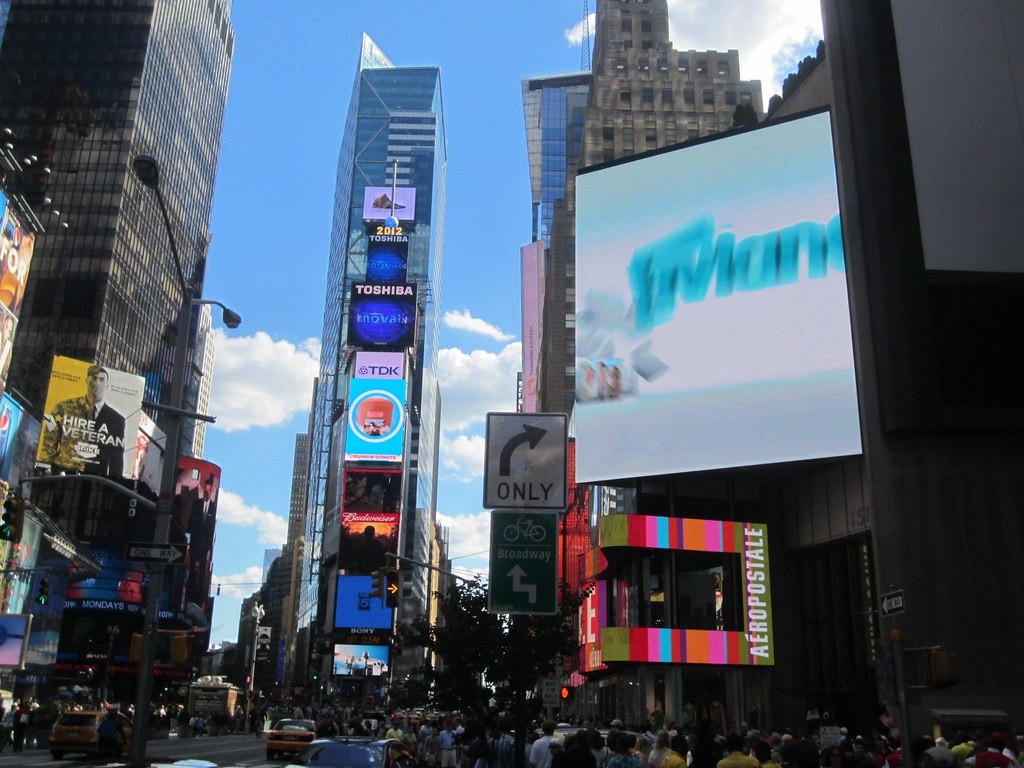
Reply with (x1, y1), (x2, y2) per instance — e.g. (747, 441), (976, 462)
(244, 602), (265, 734)
(123, 154), (240, 768)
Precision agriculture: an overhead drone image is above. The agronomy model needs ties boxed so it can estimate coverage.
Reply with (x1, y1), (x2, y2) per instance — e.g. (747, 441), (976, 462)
(88, 405), (97, 421)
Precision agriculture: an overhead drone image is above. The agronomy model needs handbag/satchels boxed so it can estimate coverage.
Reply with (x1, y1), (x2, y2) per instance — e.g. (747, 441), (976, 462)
(20, 713), (29, 726)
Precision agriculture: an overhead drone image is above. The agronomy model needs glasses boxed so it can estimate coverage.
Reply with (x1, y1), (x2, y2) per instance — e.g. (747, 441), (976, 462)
(611, 725), (617, 728)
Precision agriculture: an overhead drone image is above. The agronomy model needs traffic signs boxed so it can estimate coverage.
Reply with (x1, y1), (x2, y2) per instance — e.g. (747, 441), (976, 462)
(482, 411), (573, 514)
(882, 588), (904, 618)
(123, 541), (187, 566)
(487, 510), (560, 618)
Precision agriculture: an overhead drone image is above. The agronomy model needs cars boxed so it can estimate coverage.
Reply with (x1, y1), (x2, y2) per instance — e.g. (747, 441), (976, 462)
(266, 718), (318, 760)
(48, 710), (146, 760)
(550, 725), (646, 749)
(284, 735), (425, 768)
(27, 701), (59, 730)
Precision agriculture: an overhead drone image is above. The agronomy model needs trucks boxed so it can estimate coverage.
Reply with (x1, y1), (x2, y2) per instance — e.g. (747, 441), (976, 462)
(187, 673), (240, 725)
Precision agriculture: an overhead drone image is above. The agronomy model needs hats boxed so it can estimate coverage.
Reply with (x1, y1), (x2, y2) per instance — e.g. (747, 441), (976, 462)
(934, 737), (949, 748)
(610, 719), (623, 726)
(988, 732), (1007, 747)
(744, 729), (761, 741)
(781, 734), (793, 743)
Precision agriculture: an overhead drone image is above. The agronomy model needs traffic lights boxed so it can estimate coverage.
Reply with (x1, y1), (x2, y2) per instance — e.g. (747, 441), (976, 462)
(385, 570), (400, 608)
(0, 497), (25, 543)
(368, 566), (384, 599)
(36, 577), (50, 605)
(560, 686), (574, 701)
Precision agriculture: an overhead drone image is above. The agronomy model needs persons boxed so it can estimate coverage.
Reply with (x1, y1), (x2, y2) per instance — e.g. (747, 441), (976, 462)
(40, 365), (125, 478)
(0, 696), (1024, 768)
(187, 478), (216, 605)
